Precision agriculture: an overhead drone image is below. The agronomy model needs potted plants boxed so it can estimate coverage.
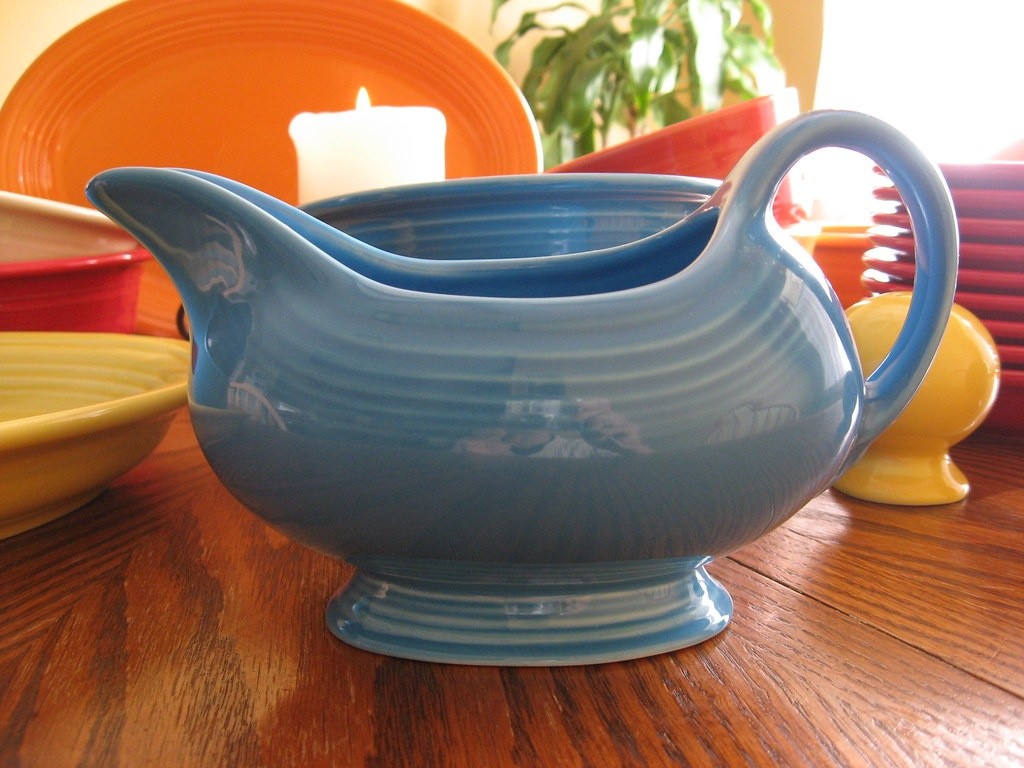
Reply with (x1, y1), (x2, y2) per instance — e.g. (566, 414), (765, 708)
(491, 1), (800, 229)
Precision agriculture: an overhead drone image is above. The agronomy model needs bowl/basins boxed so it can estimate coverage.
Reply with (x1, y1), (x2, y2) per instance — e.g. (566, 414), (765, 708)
(1, 190), (138, 260)
(0, 333), (190, 539)
(543, 85), (819, 227)
(0, 245), (155, 335)
(793, 226), (876, 310)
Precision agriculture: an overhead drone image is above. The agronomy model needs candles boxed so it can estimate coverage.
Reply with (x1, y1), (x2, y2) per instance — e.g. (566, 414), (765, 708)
(286, 83), (447, 210)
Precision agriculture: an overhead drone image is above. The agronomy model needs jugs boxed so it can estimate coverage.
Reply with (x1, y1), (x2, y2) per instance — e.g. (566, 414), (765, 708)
(85, 108), (959, 667)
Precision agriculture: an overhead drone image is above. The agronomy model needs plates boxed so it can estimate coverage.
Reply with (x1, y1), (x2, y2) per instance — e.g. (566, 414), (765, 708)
(859, 165), (1024, 430)
(0, 3), (545, 210)
